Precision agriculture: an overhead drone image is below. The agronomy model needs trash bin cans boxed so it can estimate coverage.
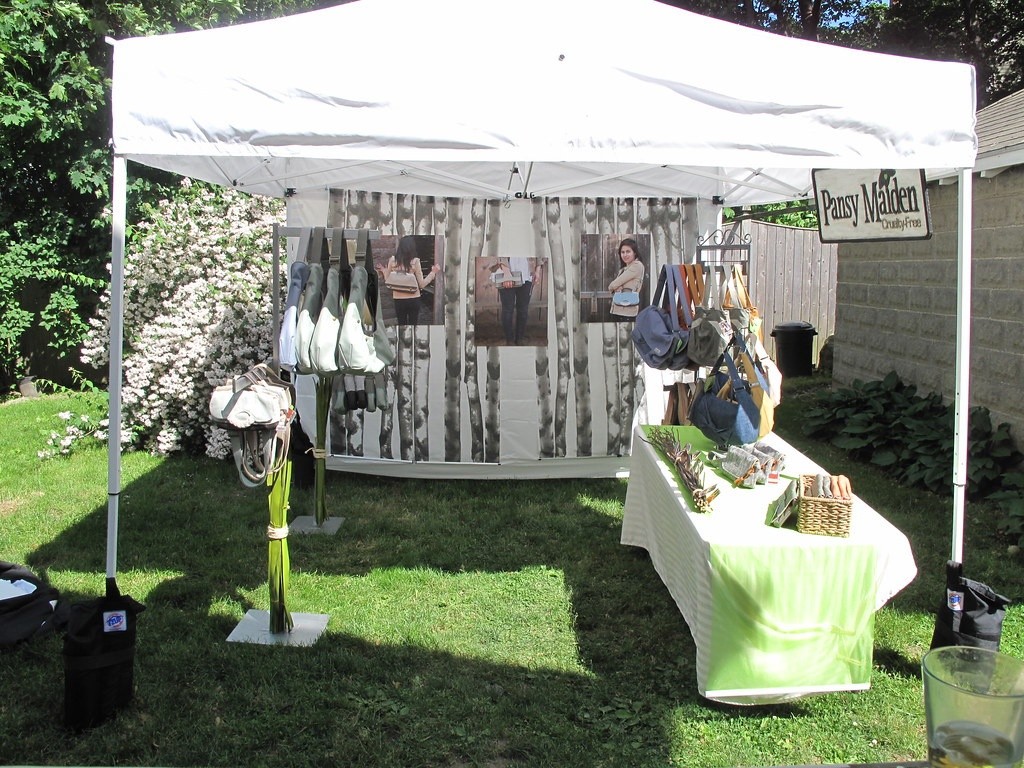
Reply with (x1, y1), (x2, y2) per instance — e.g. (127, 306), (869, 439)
(770, 321), (818, 378)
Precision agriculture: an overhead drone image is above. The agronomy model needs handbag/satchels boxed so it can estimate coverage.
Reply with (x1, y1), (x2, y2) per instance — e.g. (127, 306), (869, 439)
(722, 442), (784, 488)
(632, 264), (763, 372)
(691, 333), (782, 446)
(929, 560), (1010, 693)
(280, 226), (397, 375)
(771, 480), (797, 528)
(496, 271), (523, 288)
(210, 365), (297, 432)
(613, 291), (639, 307)
(331, 375), (389, 414)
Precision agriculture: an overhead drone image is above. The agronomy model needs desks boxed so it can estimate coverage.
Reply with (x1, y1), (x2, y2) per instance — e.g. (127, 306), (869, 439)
(618, 425), (918, 704)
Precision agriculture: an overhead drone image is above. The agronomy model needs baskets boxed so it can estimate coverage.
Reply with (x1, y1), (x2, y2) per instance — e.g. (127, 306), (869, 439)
(796, 474), (854, 538)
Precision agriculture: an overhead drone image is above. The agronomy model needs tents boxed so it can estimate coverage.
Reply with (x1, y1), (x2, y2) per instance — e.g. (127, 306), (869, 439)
(104, 0), (977, 588)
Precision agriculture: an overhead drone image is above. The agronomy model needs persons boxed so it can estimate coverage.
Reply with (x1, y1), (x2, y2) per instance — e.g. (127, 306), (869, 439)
(608, 238), (647, 322)
(488, 257), (544, 346)
(377, 236), (440, 325)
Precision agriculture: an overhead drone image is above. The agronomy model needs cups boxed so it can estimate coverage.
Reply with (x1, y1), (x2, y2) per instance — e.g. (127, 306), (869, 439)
(921, 647), (1024, 767)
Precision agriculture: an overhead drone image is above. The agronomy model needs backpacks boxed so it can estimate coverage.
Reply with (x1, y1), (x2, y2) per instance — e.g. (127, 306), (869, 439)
(386, 256), (419, 294)
(0, 560), (70, 658)
